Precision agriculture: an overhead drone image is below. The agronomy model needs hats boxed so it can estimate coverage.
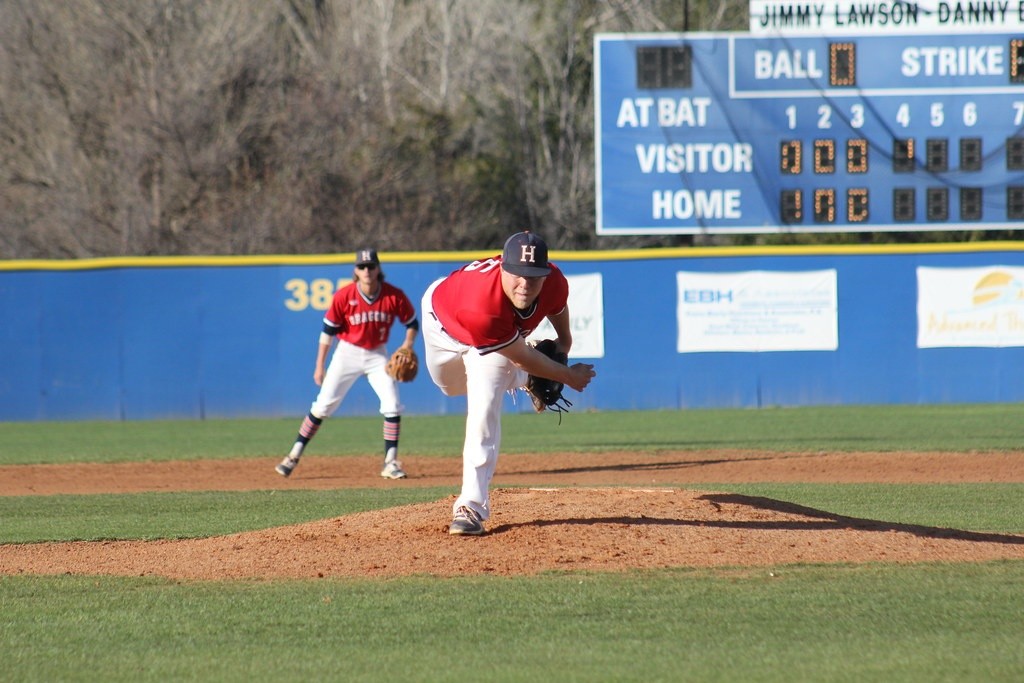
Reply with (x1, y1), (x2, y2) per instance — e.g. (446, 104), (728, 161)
(501, 230), (551, 277)
(354, 248), (379, 265)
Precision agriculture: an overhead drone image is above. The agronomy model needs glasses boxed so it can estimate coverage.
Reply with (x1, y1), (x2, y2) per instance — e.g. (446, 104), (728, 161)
(357, 264), (376, 270)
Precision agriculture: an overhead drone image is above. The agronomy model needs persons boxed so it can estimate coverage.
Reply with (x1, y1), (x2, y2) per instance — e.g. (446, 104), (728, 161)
(422, 231), (596, 535)
(275, 248), (419, 479)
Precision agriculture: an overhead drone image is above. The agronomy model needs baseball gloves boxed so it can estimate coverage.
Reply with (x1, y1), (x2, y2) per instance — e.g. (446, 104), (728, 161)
(523, 339), (573, 427)
(385, 348), (418, 382)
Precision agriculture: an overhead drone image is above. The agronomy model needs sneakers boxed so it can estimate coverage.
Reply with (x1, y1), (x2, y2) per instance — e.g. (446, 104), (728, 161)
(274, 455), (300, 478)
(448, 507), (485, 535)
(379, 459), (408, 479)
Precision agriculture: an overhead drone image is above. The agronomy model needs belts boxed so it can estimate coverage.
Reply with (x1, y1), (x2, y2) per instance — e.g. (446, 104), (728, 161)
(431, 314), (471, 347)
